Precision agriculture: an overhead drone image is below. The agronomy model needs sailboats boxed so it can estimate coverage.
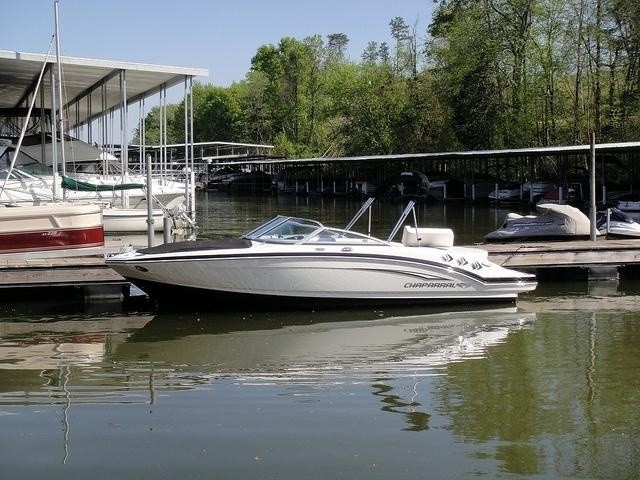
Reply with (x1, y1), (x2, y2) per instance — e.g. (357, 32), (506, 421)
(1, 1), (195, 255)
(2, 283), (162, 464)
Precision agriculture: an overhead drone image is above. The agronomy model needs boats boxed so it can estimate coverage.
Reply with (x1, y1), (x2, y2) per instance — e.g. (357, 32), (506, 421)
(484, 204), (602, 241)
(536, 189), (568, 205)
(103, 215), (539, 306)
(114, 305), (536, 383)
(597, 207), (639, 237)
(488, 188), (520, 200)
(1, 130), (192, 213)
(524, 181), (553, 197)
